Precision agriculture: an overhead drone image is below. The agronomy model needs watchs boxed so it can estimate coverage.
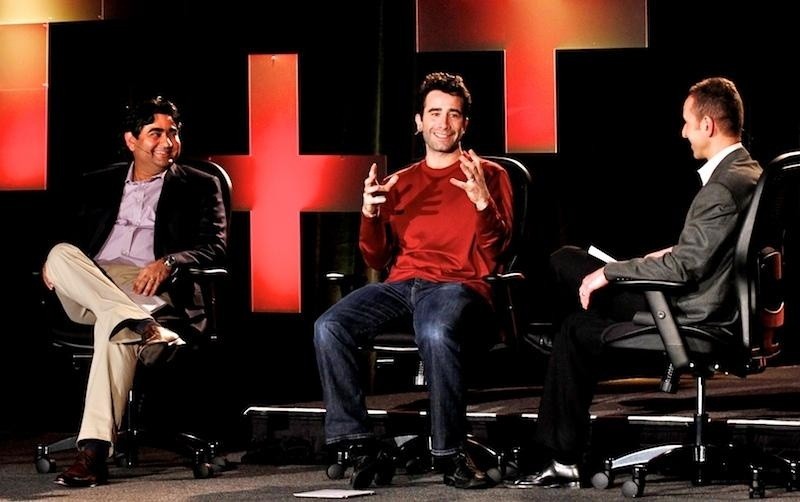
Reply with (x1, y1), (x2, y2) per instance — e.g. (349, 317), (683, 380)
(163, 254), (180, 278)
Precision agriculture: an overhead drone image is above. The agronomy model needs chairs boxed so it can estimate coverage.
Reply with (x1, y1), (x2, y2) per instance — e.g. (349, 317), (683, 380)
(319, 154), (534, 487)
(585, 152), (796, 497)
(24, 156), (243, 488)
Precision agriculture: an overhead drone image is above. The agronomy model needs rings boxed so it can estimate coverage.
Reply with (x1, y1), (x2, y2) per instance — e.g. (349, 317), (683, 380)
(580, 292), (585, 297)
(469, 176), (475, 182)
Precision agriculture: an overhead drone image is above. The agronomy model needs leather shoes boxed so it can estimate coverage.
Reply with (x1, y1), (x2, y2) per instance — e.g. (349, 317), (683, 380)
(503, 459), (581, 489)
(137, 326), (186, 370)
(522, 333), (556, 354)
(53, 455), (109, 488)
(351, 449), (384, 490)
(444, 453), (488, 489)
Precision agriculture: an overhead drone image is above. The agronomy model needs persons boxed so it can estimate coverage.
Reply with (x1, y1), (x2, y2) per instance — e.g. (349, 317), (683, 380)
(313, 70), (514, 489)
(514, 76), (764, 490)
(41, 94), (229, 488)
